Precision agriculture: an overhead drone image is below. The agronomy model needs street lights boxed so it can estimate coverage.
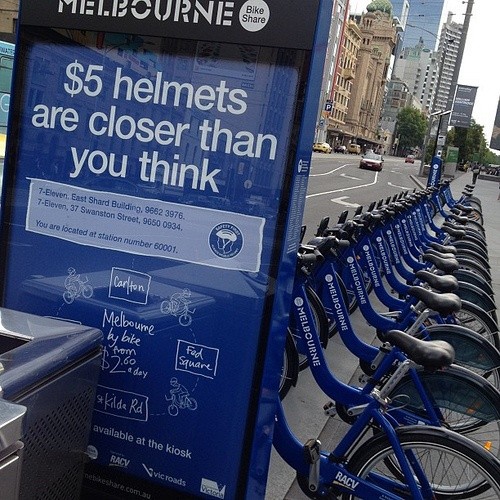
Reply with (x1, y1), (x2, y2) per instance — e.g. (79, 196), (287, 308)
(407, 23), (448, 175)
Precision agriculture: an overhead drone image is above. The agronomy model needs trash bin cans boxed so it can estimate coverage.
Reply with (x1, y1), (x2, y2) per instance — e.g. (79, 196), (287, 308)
(147, 263), (277, 320)
(0, 307), (103, 500)
(17, 270), (215, 335)
(423, 165), (431, 177)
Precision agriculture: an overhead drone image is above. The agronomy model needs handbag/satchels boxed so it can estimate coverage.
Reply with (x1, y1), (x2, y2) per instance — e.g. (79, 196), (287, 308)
(473, 169), (480, 173)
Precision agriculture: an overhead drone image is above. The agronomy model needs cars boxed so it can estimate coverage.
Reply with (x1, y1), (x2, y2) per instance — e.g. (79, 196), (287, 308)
(335, 145), (347, 154)
(359, 153), (385, 172)
(405, 155), (415, 164)
(313, 142), (332, 154)
(458, 162), (468, 172)
(349, 144), (361, 155)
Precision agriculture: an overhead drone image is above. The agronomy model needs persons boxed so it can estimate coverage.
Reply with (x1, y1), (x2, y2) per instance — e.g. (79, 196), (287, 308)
(464, 160), (500, 185)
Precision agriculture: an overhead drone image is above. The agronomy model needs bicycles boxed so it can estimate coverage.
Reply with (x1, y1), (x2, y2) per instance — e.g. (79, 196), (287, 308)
(271, 180), (500, 500)
